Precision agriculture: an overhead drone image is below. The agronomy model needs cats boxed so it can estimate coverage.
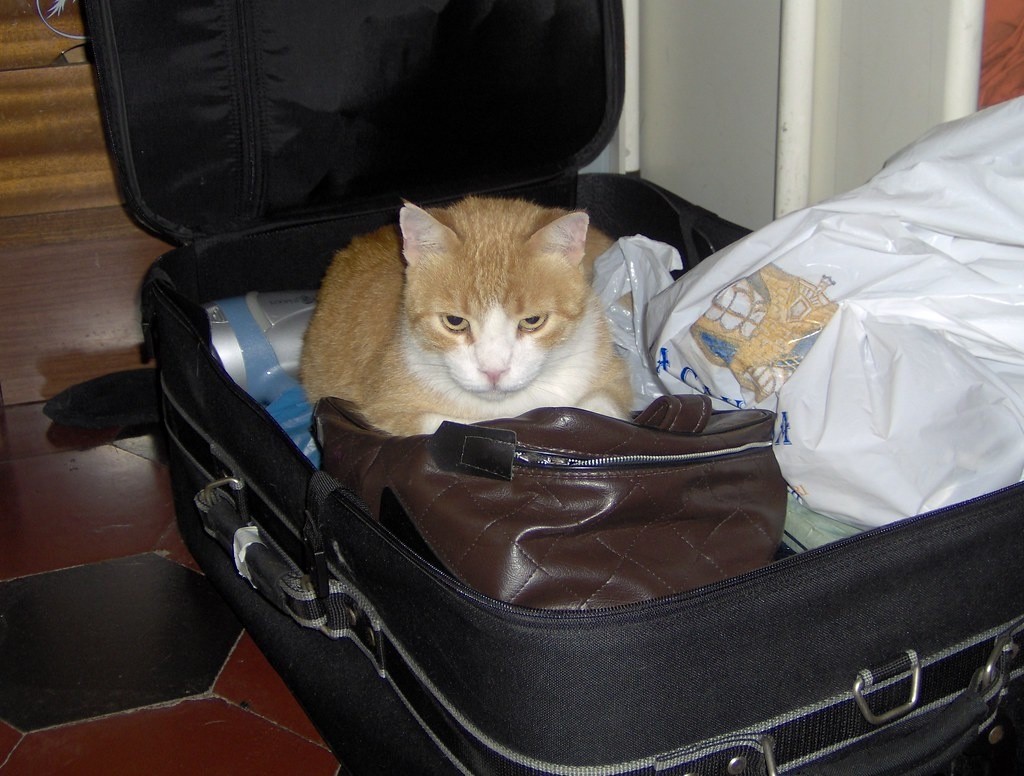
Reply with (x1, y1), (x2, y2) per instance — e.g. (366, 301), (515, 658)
(298, 195), (639, 439)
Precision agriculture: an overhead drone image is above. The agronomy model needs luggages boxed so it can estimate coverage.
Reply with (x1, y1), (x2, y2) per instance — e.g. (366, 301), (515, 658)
(42, 0), (1024, 776)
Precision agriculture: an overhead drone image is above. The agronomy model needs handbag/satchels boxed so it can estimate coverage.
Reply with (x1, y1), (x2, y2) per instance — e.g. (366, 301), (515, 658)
(309, 394), (788, 610)
(590, 94), (1024, 527)
